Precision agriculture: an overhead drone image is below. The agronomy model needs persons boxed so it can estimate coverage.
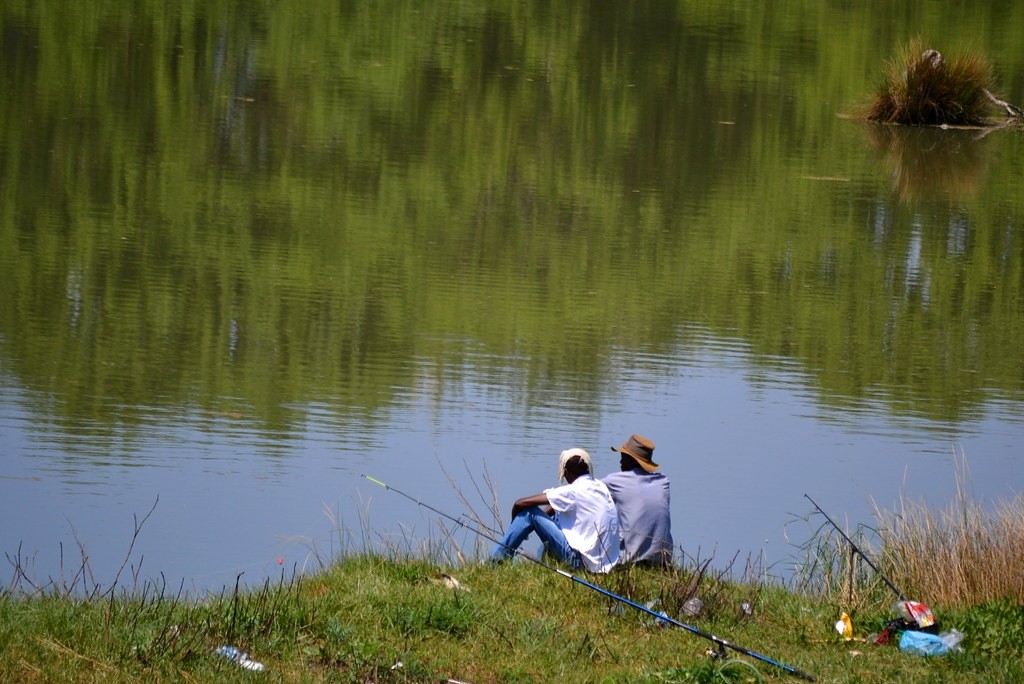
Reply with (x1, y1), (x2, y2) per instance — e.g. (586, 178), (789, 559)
(491, 449), (621, 574)
(599, 434), (672, 567)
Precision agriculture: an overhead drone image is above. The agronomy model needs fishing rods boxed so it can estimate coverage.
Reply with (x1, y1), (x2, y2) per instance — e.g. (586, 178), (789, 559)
(360, 473), (818, 682)
(803, 493), (907, 600)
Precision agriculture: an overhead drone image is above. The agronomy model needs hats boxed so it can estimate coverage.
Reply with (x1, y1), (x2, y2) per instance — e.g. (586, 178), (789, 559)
(611, 434), (660, 472)
